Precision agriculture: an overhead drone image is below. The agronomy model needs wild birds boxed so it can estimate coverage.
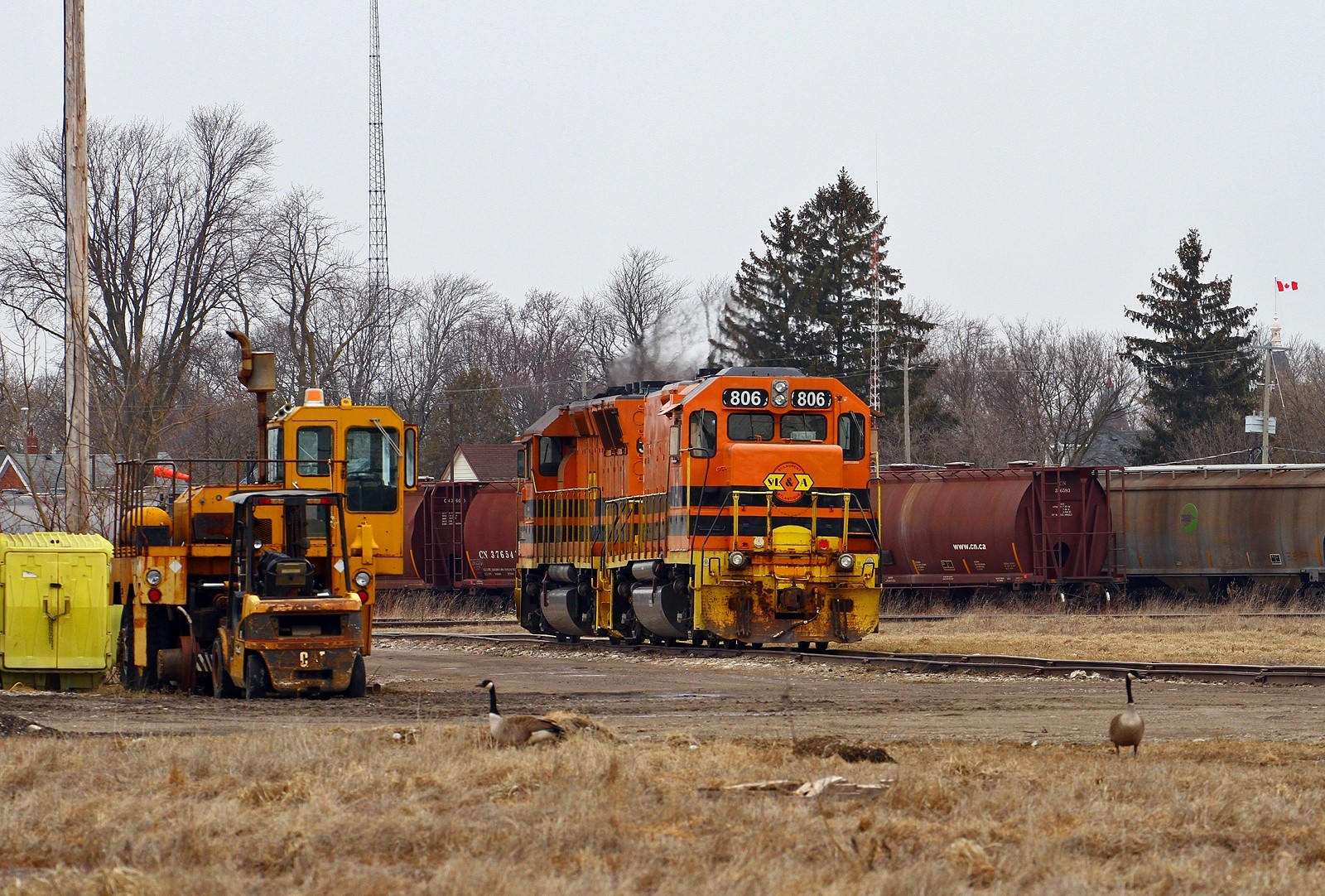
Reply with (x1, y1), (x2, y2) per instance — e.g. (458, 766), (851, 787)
(1109, 670), (1144, 759)
(475, 679), (564, 746)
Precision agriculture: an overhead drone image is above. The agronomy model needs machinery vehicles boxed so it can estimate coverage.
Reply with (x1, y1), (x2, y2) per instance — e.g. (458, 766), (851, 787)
(108, 330), (418, 699)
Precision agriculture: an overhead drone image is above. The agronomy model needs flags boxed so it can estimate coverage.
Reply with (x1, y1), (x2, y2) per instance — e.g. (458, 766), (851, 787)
(1277, 280), (1299, 292)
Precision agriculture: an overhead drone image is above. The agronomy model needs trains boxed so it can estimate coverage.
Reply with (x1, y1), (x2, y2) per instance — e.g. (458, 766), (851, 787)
(515, 367), (884, 651)
